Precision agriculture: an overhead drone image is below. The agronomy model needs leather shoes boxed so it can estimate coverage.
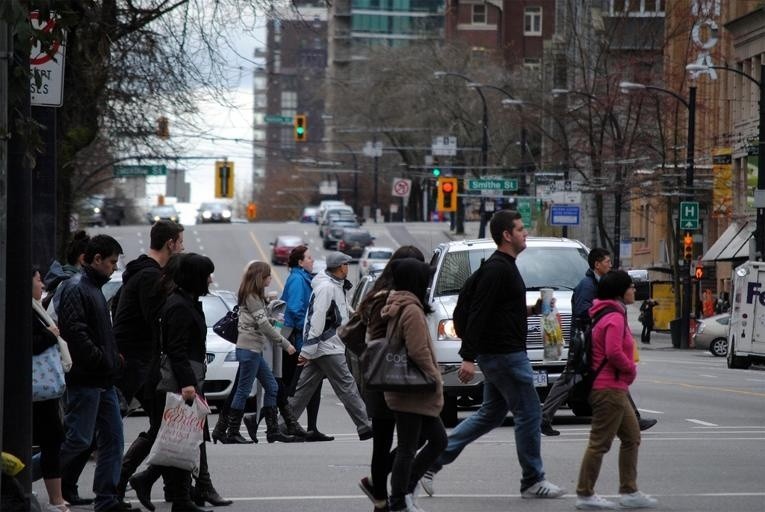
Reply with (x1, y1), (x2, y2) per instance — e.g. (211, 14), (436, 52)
(43, 435), (232, 511)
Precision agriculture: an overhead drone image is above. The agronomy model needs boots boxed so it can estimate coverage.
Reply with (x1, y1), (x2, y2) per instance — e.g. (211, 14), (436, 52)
(212, 403), (334, 444)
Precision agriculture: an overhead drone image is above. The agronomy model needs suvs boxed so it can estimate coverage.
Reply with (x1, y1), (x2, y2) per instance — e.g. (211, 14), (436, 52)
(420, 234), (605, 428)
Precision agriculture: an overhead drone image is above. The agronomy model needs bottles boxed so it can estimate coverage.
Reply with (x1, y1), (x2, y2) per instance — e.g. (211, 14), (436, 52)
(272, 312), (285, 333)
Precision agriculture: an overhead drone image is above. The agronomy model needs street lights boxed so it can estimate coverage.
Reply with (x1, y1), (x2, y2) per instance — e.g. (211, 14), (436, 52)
(680, 60), (765, 259)
(496, 95), (571, 185)
(616, 76), (700, 348)
(430, 68), (489, 178)
(462, 78), (528, 160)
(547, 82), (623, 269)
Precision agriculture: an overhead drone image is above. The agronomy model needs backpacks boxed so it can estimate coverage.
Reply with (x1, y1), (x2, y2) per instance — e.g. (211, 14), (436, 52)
(453, 255), (516, 338)
(564, 306), (626, 402)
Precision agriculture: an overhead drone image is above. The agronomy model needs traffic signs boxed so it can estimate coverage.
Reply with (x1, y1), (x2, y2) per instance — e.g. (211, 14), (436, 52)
(262, 115), (293, 124)
(467, 178), (518, 192)
(112, 163), (166, 177)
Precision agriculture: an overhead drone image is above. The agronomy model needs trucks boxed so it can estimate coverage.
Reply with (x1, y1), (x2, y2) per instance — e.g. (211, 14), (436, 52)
(723, 257), (765, 371)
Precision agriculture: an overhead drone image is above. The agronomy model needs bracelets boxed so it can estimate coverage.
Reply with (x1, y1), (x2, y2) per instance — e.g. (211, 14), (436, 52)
(532, 307), (537, 316)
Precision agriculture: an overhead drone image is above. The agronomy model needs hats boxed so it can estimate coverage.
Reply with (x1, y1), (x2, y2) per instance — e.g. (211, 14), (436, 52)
(326, 251), (353, 268)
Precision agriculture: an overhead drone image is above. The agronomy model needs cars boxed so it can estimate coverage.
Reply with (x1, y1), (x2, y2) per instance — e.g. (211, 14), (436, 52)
(198, 292), (257, 412)
(689, 311), (730, 357)
(193, 200), (232, 223)
(105, 267), (237, 326)
(147, 203), (181, 226)
(269, 235), (309, 267)
(298, 199), (406, 280)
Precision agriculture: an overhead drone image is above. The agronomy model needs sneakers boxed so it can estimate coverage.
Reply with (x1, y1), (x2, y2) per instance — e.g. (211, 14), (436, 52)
(521, 479), (567, 498)
(618, 491), (659, 509)
(357, 450), (434, 512)
(540, 417), (560, 435)
(575, 493), (618, 511)
(641, 419), (657, 430)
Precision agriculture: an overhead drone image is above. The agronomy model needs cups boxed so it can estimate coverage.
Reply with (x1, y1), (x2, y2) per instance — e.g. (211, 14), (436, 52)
(268, 291), (278, 301)
(540, 289), (553, 316)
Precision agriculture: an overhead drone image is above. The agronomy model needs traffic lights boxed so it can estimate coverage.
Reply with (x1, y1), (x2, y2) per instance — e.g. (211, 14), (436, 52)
(684, 236), (693, 261)
(293, 115), (306, 142)
(696, 267), (703, 280)
(437, 176), (457, 212)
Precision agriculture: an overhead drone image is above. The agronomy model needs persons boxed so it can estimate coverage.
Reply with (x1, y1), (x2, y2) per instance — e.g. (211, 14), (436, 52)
(640, 297), (659, 344)
(540, 248), (657, 436)
(575, 268), (658, 510)
(30, 219), (233, 512)
(414, 209), (568, 498)
(212, 246), (371, 443)
(358, 246), (449, 512)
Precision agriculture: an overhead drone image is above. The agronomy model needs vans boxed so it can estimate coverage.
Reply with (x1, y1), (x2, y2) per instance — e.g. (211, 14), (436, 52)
(78, 193), (127, 228)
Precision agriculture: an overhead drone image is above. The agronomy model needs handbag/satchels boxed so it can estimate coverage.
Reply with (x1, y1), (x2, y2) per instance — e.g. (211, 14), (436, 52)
(156, 352), (207, 396)
(338, 313), (436, 393)
(32, 343), (67, 404)
(213, 311), (239, 344)
